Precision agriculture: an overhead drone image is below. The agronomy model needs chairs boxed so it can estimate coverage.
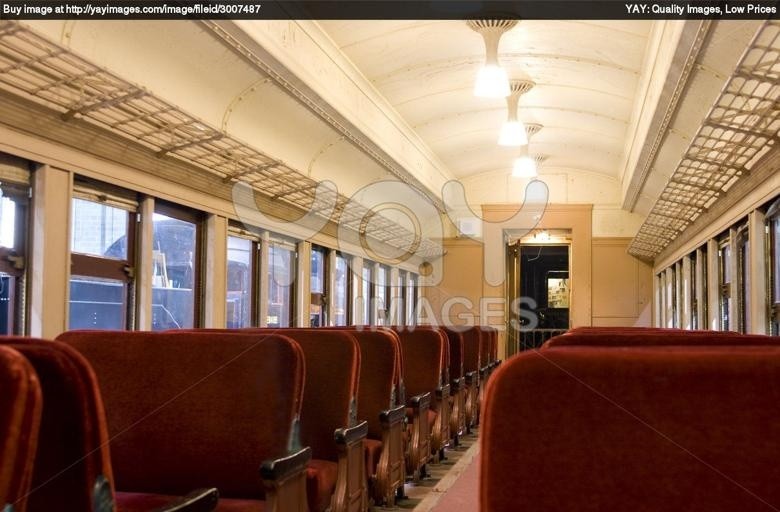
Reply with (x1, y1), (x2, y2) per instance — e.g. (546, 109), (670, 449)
(462, 328), (481, 435)
(440, 326), (465, 446)
(479, 348), (780, 510)
(413, 328), (450, 462)
(0, 346), (42, 511)
(480, 328), (488, 383)
(336, 327), (407, 507)
(493, 329), (501, 366)
(1, 337), (217, 510)
(546, 327), (778, 346)
(54, 331), (311, 512)
(488, 328), (495, 373)
(390, 326), (431, 484)
(267, 329), (368, 511)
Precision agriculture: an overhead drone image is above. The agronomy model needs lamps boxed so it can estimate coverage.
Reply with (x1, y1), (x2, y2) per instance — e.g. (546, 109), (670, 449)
(466, 21), (516, 99)
(497, 79), (535, 148)
(508, 125), (542, 179)
